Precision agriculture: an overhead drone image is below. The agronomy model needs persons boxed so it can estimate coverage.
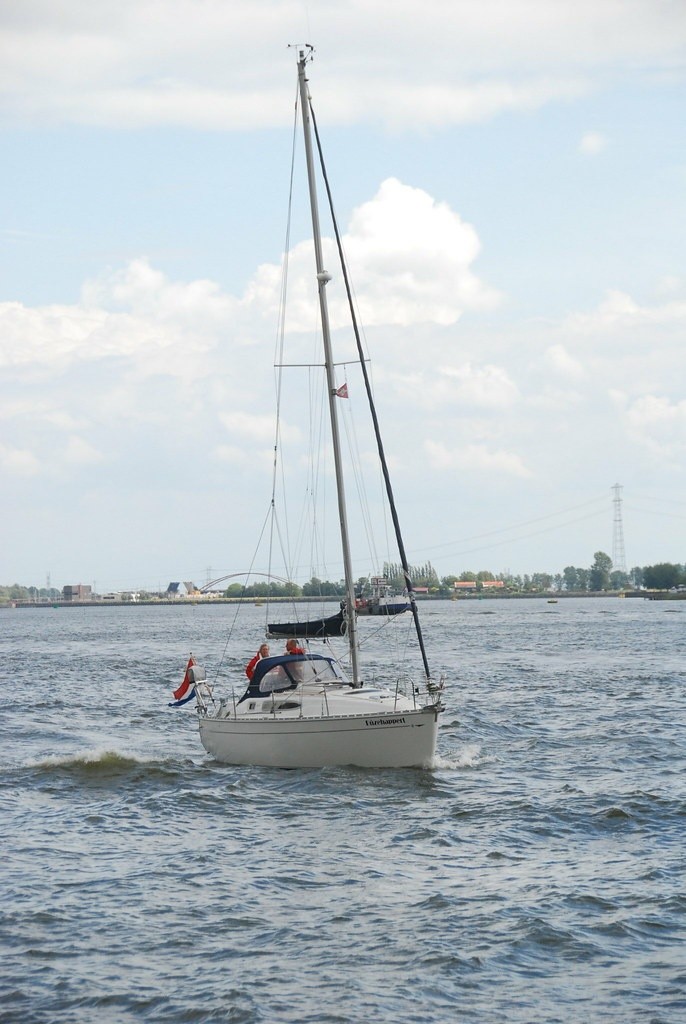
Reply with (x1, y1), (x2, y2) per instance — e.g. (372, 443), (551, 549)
(246, 643), (270, 682)
(278, 639), (306, 683)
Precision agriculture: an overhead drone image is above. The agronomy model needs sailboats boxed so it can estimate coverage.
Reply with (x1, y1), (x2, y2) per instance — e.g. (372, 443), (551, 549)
(189, 42), (444, 769)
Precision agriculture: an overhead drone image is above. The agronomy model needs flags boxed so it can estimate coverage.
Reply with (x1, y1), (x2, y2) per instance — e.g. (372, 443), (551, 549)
(168, 656), (197, 708)
